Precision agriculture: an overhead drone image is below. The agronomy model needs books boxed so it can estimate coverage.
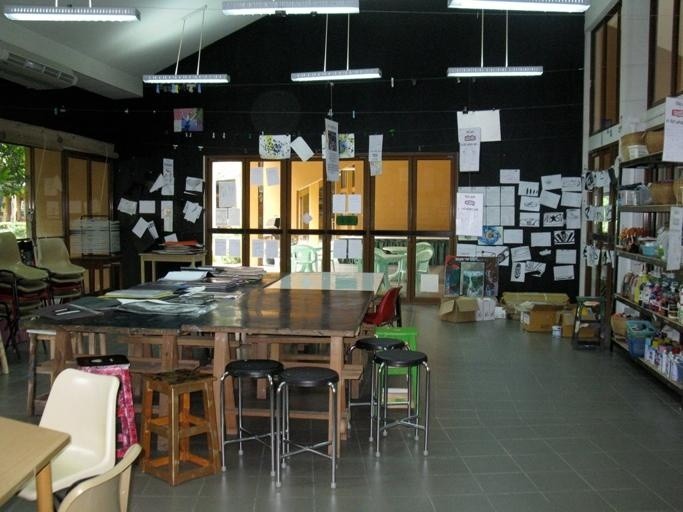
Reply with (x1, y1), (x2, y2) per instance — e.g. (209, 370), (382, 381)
(31, 264), (265, 323)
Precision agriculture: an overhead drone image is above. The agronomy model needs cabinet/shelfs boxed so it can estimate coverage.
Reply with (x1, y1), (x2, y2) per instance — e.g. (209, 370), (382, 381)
(610, 150), (683, 397)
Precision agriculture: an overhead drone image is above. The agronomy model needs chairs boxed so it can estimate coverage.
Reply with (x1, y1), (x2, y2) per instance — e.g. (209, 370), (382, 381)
(0, 232), (86, 359)
(362, 286), (402, 327)
(354, 242), (435, 293)
(57, 443), (143, 512)
(15, 367), (121, 512)
(290, 242), (317, 272)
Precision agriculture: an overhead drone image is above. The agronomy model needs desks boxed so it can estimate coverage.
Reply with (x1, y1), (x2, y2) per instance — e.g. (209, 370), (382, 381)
(18, 272), (387, 458)
(0, 417), (70, 512)
(138, 250), (207, 283)
(67, 255), (125, 302)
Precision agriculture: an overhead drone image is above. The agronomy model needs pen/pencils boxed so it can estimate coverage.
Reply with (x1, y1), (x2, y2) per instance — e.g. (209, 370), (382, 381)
(53, 307), (70, 313)
(55, 310), (82, 316)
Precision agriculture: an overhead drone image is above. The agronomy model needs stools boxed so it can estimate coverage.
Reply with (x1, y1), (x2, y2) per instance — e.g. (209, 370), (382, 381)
(371, 350), (431, 457)
(571, 296), (608, 352)
(77, 354), (339, 486)
(345, 338), (412, 457)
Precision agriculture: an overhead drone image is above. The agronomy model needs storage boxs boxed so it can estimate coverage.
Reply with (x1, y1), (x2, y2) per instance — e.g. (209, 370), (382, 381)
(439, 292), (598, 338)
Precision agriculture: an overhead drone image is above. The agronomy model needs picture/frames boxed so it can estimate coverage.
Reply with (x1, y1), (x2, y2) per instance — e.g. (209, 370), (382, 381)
(444, 255), (499, 297)
(460, 261), (485, 297)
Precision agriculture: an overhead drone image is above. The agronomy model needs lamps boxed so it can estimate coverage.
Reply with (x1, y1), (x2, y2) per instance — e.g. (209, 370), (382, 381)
(447, 0), (592, 14)
(291, 14), (383, 82)
(2, 0), (140, 22)
(142, 5), (232, 84)
(447, 9), (544, 77)
(222, 0), (360, 16)
